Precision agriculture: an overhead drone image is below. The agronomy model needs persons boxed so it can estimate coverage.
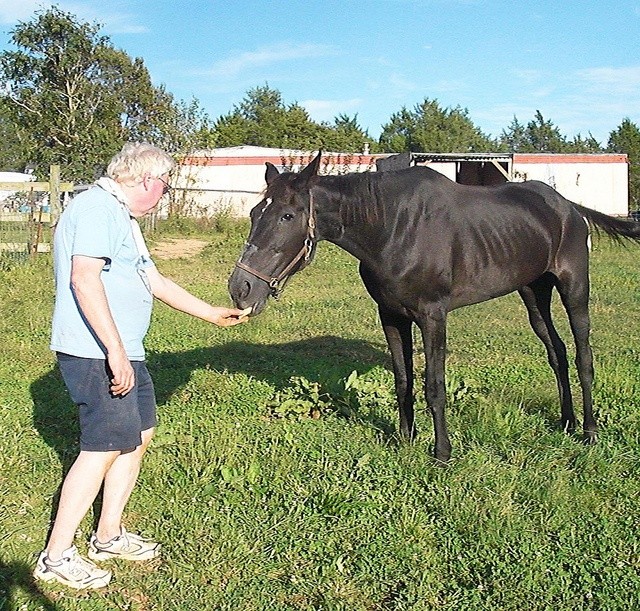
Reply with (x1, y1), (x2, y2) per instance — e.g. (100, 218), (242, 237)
(31, 144), (250, 593)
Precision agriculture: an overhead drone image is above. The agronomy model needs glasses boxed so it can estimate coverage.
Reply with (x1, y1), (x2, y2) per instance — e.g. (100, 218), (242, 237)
(158, 176), (172, 194)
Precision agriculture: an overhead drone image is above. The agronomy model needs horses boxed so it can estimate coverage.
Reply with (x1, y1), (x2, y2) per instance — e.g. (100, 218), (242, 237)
(228, 144), (640, 467)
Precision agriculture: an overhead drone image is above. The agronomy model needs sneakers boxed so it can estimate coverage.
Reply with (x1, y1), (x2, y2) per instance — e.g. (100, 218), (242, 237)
(32, 543), (113, 587)
(87, 526), (161, 561)
